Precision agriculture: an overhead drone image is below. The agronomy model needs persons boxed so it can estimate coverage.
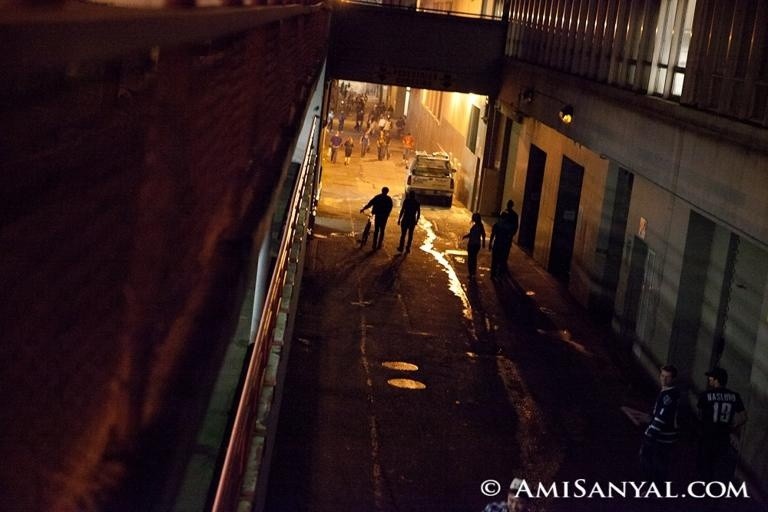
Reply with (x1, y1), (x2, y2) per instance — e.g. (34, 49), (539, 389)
(359, 186), (393, 250)
(396, 191), (421, 255)
(694, 367), (748, 481)
(329, 82), (415, 168)
(637, 364), (684, 483)
(500, 199), (519, 277)
(461, 214), (486, 277)
(488, 217), (496, 276)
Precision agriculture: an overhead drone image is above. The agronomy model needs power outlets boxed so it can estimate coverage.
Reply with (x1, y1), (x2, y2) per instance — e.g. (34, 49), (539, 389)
(559, 104), (572, 123)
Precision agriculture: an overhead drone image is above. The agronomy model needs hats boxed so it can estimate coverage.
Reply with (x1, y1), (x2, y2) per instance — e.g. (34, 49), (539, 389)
(704, 368), (728, 381)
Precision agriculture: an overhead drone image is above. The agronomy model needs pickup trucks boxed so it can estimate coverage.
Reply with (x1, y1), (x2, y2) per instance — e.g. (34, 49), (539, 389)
(405, 152), (455, 207)
